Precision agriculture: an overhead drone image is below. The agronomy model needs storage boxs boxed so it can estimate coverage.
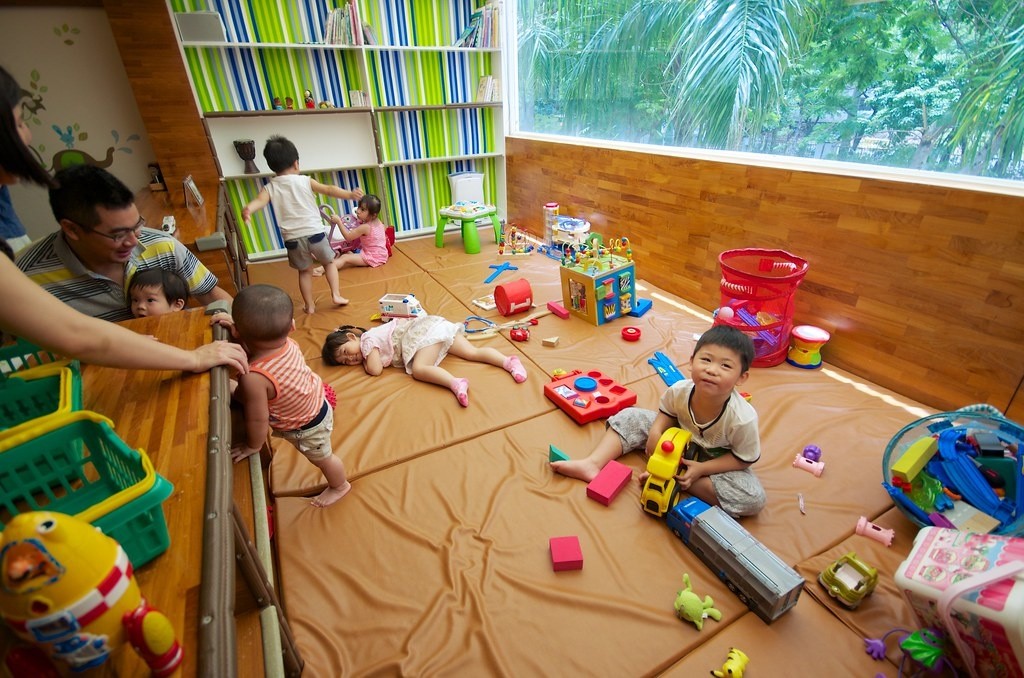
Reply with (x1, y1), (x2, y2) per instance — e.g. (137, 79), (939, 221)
(892, 525), (1024, 678)
(149, 179), (165, 192)
(0, 336), (83, 441)
(0, 411), (177, 575)
(173, 11), (228, 42)
(560, 252), (637, 326)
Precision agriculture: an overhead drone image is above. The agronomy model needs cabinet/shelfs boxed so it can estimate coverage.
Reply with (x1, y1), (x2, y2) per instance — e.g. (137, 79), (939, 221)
(137, 181), (250, 295)
(0, 299), (304, 678)
(166, 0), (507, 264)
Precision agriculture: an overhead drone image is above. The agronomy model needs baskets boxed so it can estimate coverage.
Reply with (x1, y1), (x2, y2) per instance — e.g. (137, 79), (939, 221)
(0, 337), (81, 371)
(0, 367), (86, 491)
(0, 411), (174, 573)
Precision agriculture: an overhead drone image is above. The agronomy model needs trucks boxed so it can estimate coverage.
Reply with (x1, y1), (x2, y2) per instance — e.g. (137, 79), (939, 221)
(641, 427), (700, 517)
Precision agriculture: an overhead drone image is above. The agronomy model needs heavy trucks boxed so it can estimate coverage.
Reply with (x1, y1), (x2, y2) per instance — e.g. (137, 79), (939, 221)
(666, 497), (806, 620)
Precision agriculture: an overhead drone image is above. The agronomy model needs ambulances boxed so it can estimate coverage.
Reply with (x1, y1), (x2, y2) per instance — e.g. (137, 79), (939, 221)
(378, 293), (428, 323)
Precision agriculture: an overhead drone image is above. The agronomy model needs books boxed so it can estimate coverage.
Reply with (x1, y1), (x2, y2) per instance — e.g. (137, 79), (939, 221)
(299, 0), (364, 45)
(349, 90), (368, 107)
(452, 4), (499, 48)
(475, 75), (500, 102)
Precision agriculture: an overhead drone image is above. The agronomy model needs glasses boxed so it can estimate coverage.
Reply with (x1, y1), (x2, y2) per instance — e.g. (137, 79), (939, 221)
(69, 216), (145, 242)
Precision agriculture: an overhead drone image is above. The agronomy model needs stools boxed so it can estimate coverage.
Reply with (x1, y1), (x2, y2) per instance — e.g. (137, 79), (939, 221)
(435, 198), (502, 254)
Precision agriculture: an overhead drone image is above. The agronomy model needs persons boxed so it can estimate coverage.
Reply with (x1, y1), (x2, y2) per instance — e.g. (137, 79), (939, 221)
(14, 163), (234, 323)
(128, 267), (190, 318)
(312, 195), (388, 277)
(274, 97), (293, 110)
(304, 90), (315, 109)
(550, 325), (766, 517)
(0, 65), (249, 376)
(241, 136), (364, 313)
(321, 315), (527, 407)
(320, 103), (327, 108)
(209, 284), (352, 507)
